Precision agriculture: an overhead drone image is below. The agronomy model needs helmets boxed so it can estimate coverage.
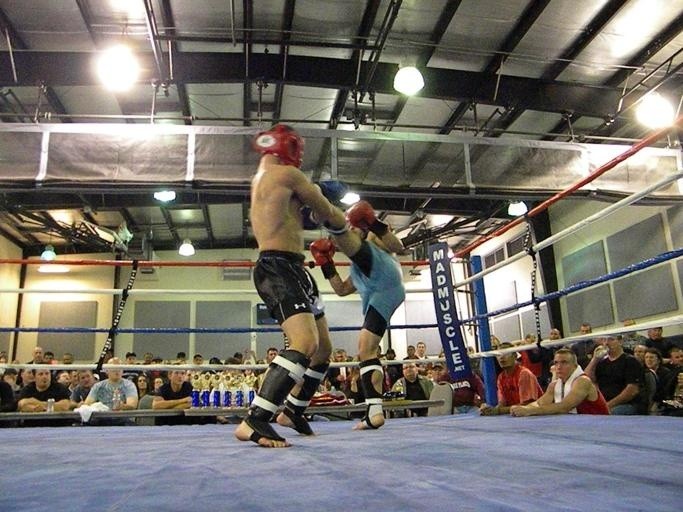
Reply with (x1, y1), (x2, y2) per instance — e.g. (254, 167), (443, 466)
(253, 125), (304, 169)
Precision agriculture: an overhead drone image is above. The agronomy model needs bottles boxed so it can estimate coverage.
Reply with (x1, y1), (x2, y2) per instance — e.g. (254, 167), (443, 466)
(112, 389), (121, 410)
(595, 346), (609, 359)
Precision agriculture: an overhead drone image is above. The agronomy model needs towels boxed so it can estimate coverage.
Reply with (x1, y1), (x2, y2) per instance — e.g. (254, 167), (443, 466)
(552, 365), (585, 414)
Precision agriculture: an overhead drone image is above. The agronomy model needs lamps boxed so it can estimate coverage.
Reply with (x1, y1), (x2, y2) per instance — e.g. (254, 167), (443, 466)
(508, 198), (528, 218)
(178, 219), (196, 257)
(40, 227), (56, 261)
(393, 38), (425, 96)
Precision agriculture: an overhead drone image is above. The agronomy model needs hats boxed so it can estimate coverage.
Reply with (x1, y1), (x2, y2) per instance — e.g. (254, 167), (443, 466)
(152, 356), (162, 362)
(433, 363), (444, 369)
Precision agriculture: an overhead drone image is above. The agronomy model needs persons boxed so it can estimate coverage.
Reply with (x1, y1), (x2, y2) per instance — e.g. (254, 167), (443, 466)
(312, 175), (405, 429)
(233, 123), (348, 448)
(302, 318), (682, 422)
(0, 347), (285, 425)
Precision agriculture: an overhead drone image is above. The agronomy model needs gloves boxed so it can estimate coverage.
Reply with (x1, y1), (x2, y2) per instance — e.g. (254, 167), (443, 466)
(348, 201), (387, 241)
(319, 178), (344, 206)
(310, 239), (337, 278)
